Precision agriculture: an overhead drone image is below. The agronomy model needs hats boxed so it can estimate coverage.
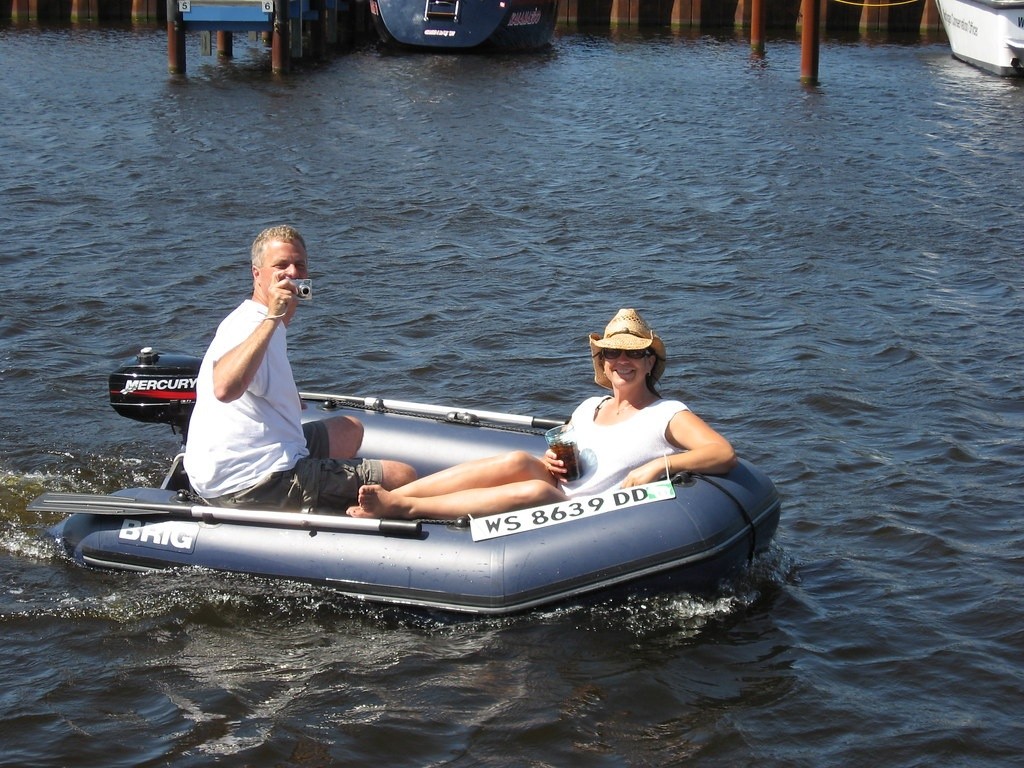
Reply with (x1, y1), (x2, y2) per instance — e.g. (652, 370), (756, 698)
(589, 309), (667, 389)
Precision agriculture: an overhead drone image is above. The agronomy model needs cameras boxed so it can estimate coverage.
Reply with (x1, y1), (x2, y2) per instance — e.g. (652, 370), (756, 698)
(284, 279), (312, 300)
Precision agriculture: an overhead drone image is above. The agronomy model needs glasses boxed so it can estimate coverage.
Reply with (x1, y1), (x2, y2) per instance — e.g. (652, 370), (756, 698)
(599, 348), (652, 359)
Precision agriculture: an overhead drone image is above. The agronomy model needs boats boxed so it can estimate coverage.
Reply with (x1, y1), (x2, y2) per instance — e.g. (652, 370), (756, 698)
(369, 0), (560, 55)
(934, 0), (1024, 80)
(60, 346), (784, 616)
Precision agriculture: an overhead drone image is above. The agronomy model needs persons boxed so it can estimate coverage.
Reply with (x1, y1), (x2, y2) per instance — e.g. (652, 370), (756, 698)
(184, 225), (418, 509)
(347, 309), (737, 518)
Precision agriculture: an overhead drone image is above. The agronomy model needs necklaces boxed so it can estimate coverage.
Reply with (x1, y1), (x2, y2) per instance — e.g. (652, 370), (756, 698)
(612, 397), (646, 414)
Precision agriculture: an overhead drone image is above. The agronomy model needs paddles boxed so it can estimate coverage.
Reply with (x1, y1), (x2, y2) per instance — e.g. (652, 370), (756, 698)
(294, 386), (578, 434)
(23, 487), (429, 543)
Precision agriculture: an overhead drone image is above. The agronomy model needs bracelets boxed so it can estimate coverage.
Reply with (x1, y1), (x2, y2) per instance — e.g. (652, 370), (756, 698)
(666, 456), (671, 477)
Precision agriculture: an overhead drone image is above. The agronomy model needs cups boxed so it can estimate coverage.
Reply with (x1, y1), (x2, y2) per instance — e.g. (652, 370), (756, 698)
(545, 425), (582, 482)
